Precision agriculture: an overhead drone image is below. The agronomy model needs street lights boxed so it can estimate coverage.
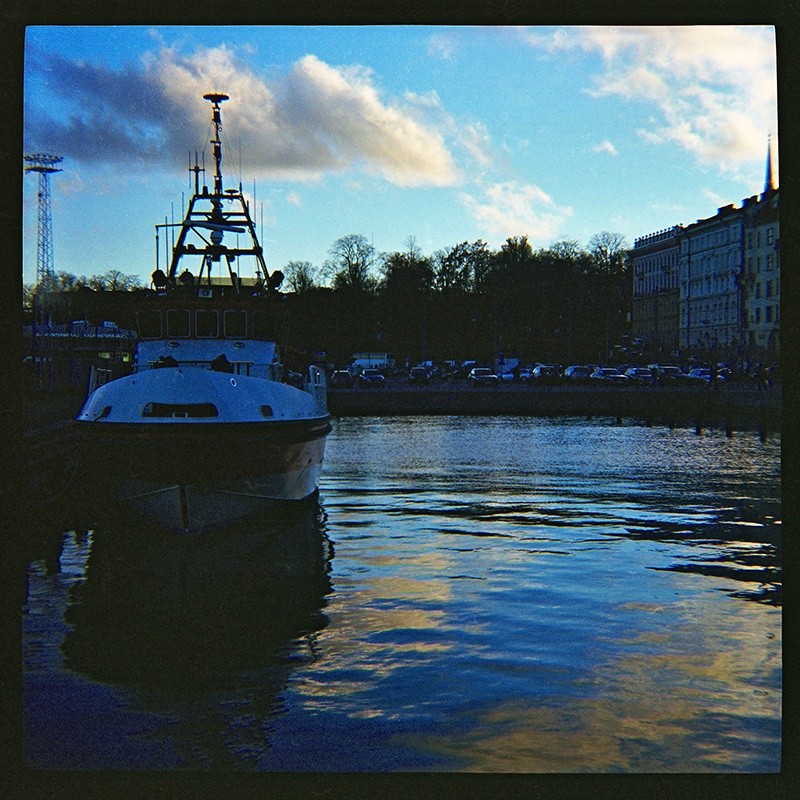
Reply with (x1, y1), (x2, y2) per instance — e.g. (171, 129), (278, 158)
(671, 225), (693, 350)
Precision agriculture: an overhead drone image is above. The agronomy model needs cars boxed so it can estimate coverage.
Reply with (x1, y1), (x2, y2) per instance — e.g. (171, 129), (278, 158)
(408, 367), (432, 386)
(466, 368), (498, 386)
(330, 370), (353, 388)
(359, 369), (385, 387)
(425, 363), (476, 383)
(499, 363), (781, 388)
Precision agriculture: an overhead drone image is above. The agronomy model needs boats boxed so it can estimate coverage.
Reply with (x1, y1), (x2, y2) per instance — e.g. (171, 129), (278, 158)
(66, 93), (335, 541)
